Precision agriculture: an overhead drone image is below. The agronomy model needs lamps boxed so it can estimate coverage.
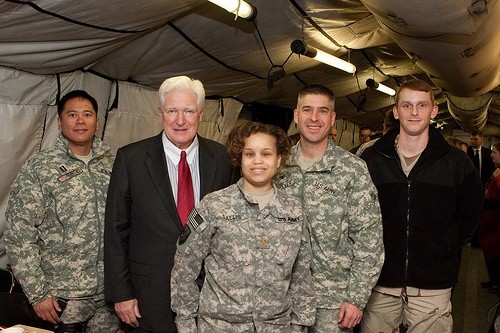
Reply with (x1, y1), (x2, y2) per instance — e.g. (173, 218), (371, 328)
(208, 0), (258, 22)
(366, 72), (397, 97)
(290, 19), (357, 75)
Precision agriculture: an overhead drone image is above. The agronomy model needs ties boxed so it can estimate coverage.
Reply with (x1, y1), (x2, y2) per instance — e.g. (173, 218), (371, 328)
(176, 151), (195, 227)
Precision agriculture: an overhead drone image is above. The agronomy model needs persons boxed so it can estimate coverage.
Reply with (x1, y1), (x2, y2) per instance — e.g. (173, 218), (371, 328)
(360, 80), (479, 333)
(4, 90), (116, 333)
(287, 108), (495, 204)
(479, 142), (499, 286)
(105, 72), (243, 333)
(289, 84), (386, 333)
(171, 123), (316, 333)
